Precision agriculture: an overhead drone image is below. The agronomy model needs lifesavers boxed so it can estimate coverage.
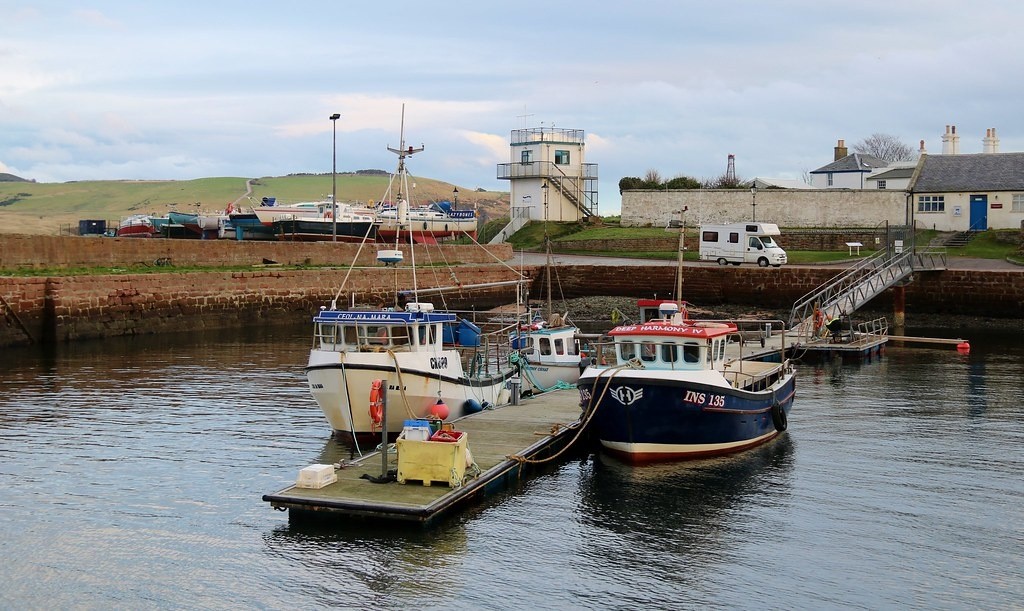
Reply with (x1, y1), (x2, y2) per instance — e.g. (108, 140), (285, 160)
(227, 202), (233, 213)
(521, 321), (545, 331)
(369, 378), (382, 424)
(813, 310), (824, 328)
(771, 401), (787, 433)
(368, 199), (374, 207)
(324, 211), (332, 218)
(681, 307), (688, 319)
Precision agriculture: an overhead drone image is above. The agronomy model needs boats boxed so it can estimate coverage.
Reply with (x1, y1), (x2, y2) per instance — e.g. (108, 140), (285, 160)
(574, 204), (798, 469)
(112, 192), (479, 244)
(509, 230), (600, 395)
(301, 100), (522, 451)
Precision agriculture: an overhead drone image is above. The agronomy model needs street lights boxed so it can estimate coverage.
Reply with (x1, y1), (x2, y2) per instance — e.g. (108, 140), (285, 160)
(451, 185), (459, 212)
(329, 112), (340, 242)
(542, 181), (551, 239)
(749, 180), (757, 223)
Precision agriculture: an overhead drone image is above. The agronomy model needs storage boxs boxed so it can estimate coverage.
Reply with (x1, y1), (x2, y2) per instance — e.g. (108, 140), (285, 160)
(297, 464), (337, 489)
(395, 420), (468, 488)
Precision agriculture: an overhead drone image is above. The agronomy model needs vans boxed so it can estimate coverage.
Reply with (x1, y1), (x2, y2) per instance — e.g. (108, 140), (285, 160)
(699, 220), (788, 267)
